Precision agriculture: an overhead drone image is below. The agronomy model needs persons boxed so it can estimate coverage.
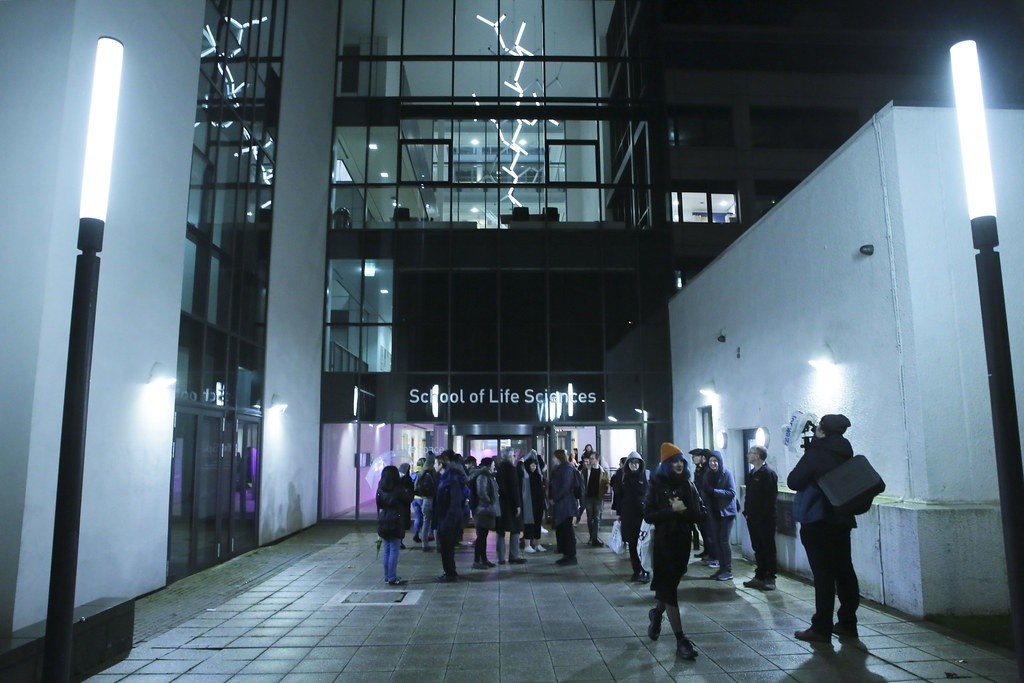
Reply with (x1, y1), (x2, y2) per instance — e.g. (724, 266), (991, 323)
(611, 451), (651, 582)
(374, 448), (477, 585)
(687, 448), (736, 580)
(568, 443), (609, 548)
(643, 442), (701, 659)
(548, 448), (579, 564)
(741, 447), (781, 590)
(466, 445), (549, 569)
(786, 413), (862, 645)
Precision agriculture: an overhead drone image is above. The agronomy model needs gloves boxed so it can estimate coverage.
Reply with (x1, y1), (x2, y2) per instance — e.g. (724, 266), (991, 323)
(703, 485), (713, 494)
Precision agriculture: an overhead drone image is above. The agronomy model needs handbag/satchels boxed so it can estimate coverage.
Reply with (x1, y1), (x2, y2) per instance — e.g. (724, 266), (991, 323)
(609, 520), (626, 554)
(817, 454), (885, 515)
(637, 518), (656, 574)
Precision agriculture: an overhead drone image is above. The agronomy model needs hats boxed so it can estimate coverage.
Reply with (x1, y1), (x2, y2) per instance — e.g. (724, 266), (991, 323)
(821, 413), (851, 434)
(689, 448), (702, 455)
(660, 443), (683, 463)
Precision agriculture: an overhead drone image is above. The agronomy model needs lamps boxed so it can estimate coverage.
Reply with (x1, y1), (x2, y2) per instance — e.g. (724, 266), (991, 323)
(715, 432), (727, 450)
(755, 427), (770, 449)
(144, 362), (177, 388)
(269, 393), (289, 411)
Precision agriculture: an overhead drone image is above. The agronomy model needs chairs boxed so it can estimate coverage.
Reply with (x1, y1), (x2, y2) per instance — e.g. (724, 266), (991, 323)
(512, 207), (529, 221)
(542, 206), (560, 222)
(393, 207), (410, 221)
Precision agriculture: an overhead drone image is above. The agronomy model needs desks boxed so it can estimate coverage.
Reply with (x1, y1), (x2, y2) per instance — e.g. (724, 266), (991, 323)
(499, 213), (545, 224)
(390, 216), (433, 221)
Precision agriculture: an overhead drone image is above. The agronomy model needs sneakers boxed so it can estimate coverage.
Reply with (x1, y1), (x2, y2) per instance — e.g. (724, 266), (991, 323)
(648, 608), (664, 640)
(711, 571), (733, 580)
(676, 637), (698, 658)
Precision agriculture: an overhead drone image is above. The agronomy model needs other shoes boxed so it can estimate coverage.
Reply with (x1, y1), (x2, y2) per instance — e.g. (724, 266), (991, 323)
(795, 628), (831, 642)
(588, 536), (604, 546)
(693, 552), (706, 558)
(831, 623), (858, 636)
(557, 557), (575, 565)
(631, 573), (650, 582)
(386, 577), (407, 585)
(743, 577), (775, 589)
(708, 560), (720, 568)
(422, 542), (547, 583)
